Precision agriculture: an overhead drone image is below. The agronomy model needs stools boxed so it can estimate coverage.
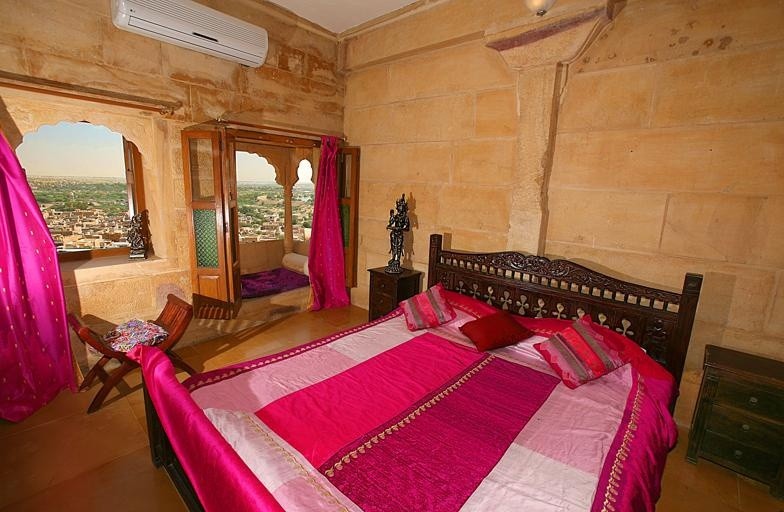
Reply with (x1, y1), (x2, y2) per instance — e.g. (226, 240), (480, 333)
(67, 294), (197, 414)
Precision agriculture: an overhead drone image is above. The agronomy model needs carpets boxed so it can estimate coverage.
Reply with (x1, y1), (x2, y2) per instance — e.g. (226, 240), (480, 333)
(240, 268), (310, 299)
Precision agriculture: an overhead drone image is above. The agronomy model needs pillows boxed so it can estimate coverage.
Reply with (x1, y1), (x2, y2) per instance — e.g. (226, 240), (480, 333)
(281, 252), (309, 276)
(398, 281), (457, 331)
(533, 313), (634, 390)
(458, 310), (536, 352)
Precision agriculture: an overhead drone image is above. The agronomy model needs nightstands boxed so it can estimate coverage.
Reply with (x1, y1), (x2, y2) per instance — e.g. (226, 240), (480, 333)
(685, 344), (784, 501)
(367, 266), (422, 322)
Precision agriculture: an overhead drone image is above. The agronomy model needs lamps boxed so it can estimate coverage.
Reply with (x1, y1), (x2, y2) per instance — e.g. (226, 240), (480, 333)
(522, 0), (557, 17)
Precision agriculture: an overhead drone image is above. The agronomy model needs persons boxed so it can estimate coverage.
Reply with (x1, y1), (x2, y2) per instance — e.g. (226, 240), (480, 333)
(384, 193), (409, 267)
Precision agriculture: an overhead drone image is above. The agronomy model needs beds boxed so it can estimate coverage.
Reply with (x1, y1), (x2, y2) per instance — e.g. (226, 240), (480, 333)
(140, 234), (703, 512)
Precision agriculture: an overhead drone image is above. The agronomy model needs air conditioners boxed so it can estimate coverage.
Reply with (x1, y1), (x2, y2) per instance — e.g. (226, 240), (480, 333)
(110, 0), (268, 68)
